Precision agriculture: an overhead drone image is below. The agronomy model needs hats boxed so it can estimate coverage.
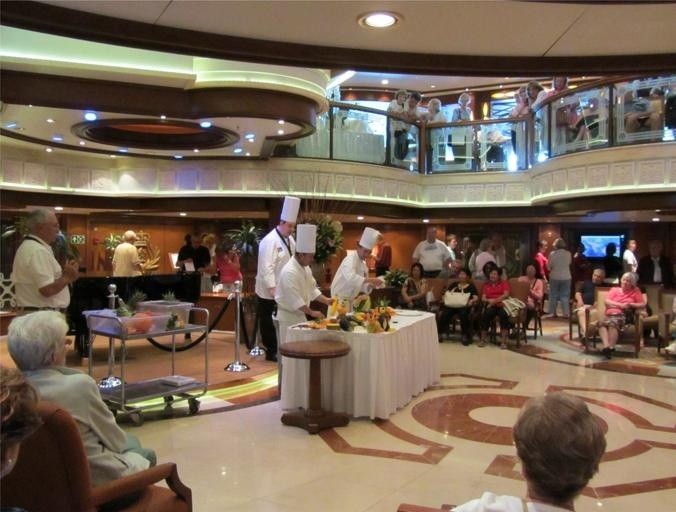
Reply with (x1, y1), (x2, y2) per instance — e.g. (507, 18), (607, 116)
(280, 196), (301, 223)
(358, 227), (379, 250)
(296, 224), (317, 254)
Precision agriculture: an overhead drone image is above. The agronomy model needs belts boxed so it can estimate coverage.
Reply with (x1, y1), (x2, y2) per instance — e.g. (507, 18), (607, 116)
(17, 307), (65, 314)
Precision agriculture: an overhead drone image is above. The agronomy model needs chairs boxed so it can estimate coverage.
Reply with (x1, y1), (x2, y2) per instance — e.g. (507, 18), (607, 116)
(2, 402), (194, 512)
(415, 276), (675, 362)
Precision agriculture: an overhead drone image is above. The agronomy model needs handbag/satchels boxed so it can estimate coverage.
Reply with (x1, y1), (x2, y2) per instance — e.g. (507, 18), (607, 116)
(501, 296), (526, 318)
(626, 307), (635, 324)
(443, 287), (471, 309)
(425, 287), (441, 312)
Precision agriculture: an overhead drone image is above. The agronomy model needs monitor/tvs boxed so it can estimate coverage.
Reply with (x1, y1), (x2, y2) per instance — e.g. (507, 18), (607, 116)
(577, 232), (626, 261)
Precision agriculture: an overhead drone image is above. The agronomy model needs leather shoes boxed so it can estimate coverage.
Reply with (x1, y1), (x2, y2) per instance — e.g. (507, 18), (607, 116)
(603, 348), (612, 360)
(602, 346), (616, 353)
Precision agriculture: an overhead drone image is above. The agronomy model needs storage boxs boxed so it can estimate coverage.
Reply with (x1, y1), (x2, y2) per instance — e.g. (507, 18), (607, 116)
(136, 300), (193, 330)
(81, 308), (171, 335)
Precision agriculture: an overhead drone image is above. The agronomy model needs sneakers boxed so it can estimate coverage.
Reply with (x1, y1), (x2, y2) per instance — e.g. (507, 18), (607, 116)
(478, 340), (487, 347)
(501, 340), (508, 348)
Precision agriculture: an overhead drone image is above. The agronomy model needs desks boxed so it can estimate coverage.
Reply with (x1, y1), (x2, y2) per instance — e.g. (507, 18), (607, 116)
(279, 307), (440, 422)
(278, 340), (352, 435)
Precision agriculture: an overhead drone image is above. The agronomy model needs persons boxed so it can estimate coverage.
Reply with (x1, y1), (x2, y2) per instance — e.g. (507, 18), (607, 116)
(272, 224), (335, 397)
(385, 72), (676, 173)
(6, 309), (159, 511)
(400, 226), (676, 359)
(367, 233), (392, 278)
(326, 226), (383, 319)
(0, 364), (43, 482)
(254, 195), (302, 362)
(11, 208), (80, 315)
(448, 391), (607, 512)
(175, 232), (244, 294)
(112, 230), (143, 277)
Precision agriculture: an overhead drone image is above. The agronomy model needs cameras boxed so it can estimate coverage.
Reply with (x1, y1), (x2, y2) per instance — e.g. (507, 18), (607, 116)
(68, 255), (87, 272)
